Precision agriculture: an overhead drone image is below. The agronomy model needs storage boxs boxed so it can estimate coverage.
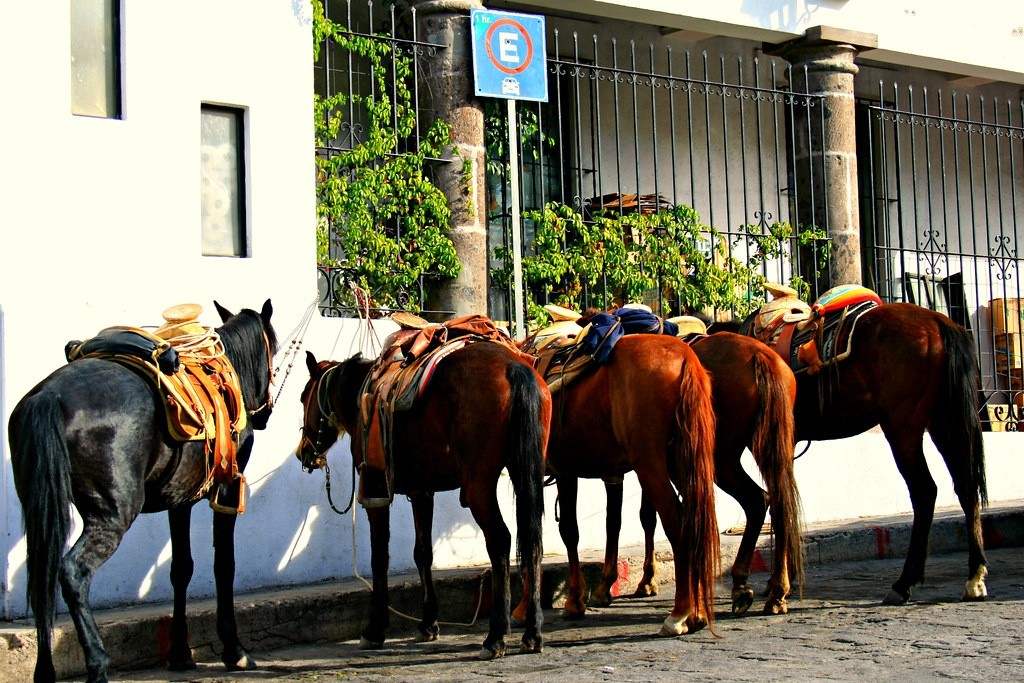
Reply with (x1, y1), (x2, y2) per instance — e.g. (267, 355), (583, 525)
(995, 334), (1024, 371)
(1008, 369), (1024, 406)
(988, 297), (1024, 335)
(987, 404), (1018, 431)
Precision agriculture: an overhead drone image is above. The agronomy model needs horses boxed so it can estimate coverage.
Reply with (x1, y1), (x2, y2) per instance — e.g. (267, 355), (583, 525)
(510, 334), (722, 637)
(0, 298), (277, 683)
(576, 304), (808, 617)
(704, 303), (988, 604)
(296, 341), (552, 659)
